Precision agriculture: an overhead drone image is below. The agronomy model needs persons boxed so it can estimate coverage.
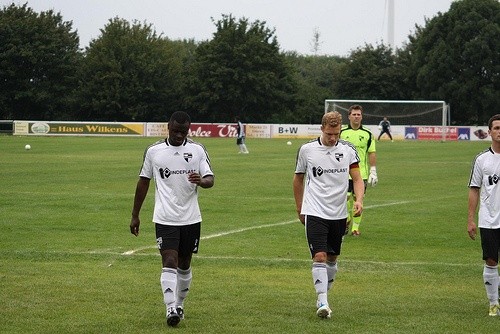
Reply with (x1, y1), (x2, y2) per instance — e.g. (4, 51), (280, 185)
(233, 116), (249, 155)
(338, 104), (379, 237)
(467, 114), (500, 316)
(293, 112), (364, 318)
(129, 110), (214, 327)
(375, 118), (392, 142)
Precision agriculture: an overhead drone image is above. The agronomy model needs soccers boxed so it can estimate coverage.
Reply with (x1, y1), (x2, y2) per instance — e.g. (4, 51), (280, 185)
(287, 141), (292, 146)
(25, 145), (31, 150)
